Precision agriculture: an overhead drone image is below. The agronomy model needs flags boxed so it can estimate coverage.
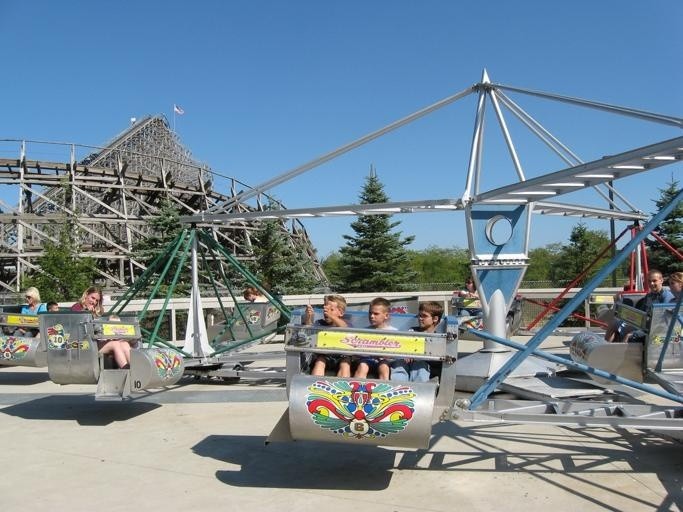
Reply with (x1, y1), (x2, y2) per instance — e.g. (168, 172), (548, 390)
(174, 104), (184, 115)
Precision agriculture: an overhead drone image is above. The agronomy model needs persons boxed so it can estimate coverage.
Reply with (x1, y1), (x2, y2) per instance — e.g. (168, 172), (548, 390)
(459, 276), (483, 315)
(305, 296), (353, 377)
(623, 271), (683, 343)
(605, 266), (674, 342)
(243, 288), (267, 302)
(71, 286), (132, 369)
(353, 296), (397, 381)
(14, 287), (47, 336)
(47, 302), (58, 312)
(390, 301), (444, 382)
